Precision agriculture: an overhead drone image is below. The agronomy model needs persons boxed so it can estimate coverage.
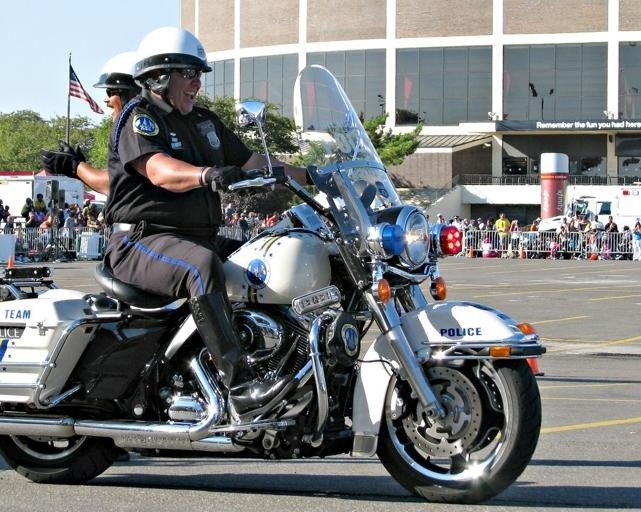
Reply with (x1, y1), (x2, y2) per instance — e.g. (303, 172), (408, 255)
(220, 201), (286, 242)
(425, 211), (640, 262)
(0, 192), (104, 263)
(100, 27), (341, 425)
(39, 50), (142, 198)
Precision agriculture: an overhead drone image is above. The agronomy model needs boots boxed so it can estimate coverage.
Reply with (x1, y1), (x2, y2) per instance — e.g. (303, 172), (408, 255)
(185, 292), (300, 425)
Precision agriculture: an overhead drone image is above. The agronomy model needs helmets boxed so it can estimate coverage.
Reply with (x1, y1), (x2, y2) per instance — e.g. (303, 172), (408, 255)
(90, 50), (142, 94)
(130, 24), (214, 80)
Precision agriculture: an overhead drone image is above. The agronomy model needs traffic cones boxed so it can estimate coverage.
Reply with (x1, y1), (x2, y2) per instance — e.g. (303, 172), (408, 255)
(6, 255), (16, 268)
(518, 246), (526, 259)
(468, 246), (475, 259)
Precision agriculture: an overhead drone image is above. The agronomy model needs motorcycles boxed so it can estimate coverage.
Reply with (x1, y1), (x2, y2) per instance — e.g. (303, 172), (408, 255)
(0, 64), (546, 505)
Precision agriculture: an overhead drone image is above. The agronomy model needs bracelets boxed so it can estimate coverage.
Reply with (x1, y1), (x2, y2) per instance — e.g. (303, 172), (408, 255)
(197, 164), (208, 188)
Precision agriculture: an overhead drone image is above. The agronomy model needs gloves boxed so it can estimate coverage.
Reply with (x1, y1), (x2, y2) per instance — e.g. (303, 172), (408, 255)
(38, 139), (86, 179)
(303, 163), (341, 199)
(203, 164), (248, 195)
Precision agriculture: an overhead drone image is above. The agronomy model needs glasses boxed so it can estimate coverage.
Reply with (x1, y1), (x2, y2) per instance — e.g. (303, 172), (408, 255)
(168, 67), (203, 80)
(104, 88), (122, 98)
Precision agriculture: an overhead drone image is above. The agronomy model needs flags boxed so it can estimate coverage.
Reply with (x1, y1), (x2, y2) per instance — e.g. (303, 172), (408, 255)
(68, 58), (104, 114)
